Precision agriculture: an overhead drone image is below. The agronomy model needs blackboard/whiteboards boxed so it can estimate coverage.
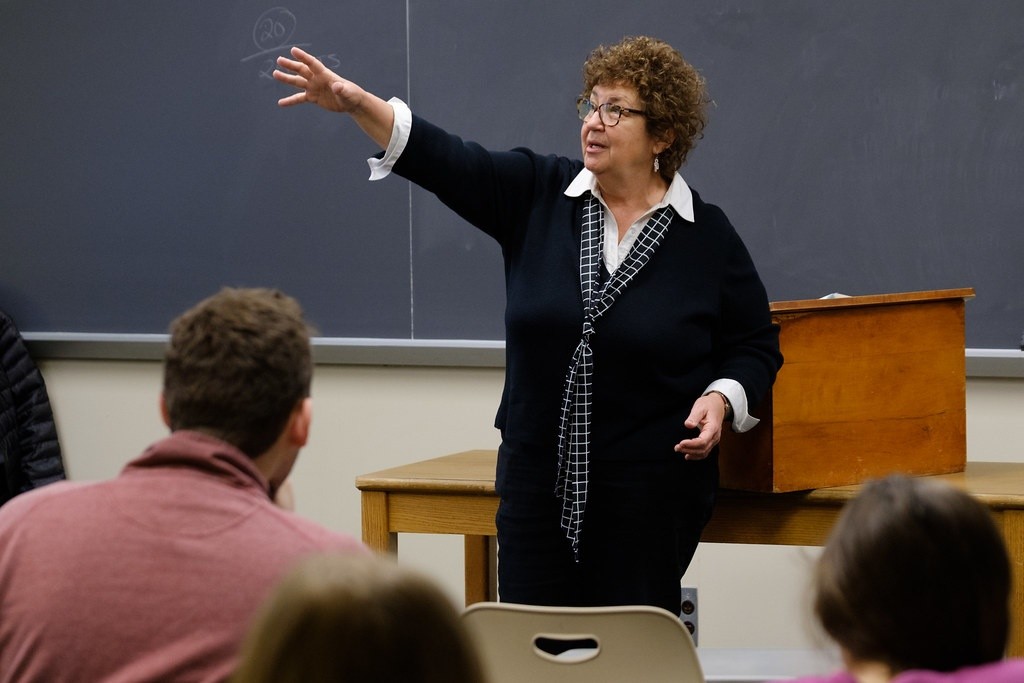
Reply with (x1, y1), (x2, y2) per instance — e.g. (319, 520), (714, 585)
(0, 0), (1024, 379)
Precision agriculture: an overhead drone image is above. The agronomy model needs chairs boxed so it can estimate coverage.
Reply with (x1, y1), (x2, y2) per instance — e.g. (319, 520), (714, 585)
(458, 601), (706, 683)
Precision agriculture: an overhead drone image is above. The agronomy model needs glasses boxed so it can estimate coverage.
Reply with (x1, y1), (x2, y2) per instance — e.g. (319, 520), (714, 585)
(575, 96), (647, 127)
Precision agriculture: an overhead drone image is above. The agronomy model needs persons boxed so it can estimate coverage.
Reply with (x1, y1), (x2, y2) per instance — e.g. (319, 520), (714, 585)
(269, 34), (784, 621)
(1, 287), (68, 512)
(1, 288), (372, 683)
(240, 553), (491, 682)
(812, 473), (1023, 683)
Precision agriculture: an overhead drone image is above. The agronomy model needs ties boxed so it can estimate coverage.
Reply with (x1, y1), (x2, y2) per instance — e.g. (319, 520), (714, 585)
(553, 190), (676, 563)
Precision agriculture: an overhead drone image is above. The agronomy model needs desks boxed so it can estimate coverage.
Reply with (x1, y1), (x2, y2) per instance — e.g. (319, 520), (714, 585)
(357, 448), (1024, 663)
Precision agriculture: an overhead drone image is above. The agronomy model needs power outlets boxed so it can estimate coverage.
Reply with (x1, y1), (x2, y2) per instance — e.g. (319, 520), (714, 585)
(678, 587), (698, 648)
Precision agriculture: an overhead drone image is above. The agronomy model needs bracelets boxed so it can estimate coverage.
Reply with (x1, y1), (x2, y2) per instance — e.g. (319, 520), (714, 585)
(717, 391), (733, 419)
